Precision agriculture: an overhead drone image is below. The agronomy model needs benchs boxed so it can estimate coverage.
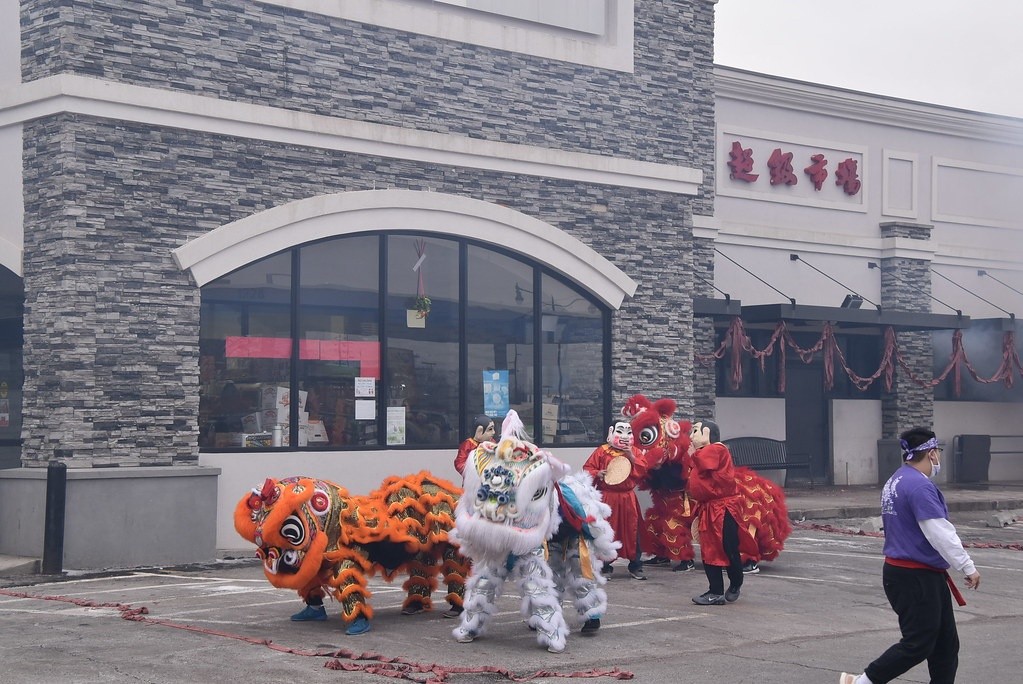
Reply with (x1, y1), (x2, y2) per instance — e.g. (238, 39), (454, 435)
(720, 436), (816, 490)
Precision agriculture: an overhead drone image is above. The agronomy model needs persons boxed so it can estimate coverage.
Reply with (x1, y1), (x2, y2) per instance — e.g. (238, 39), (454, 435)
(683, 418), (747, 605)
(838, 422), (982, 684)
(454, 415), (504, 489)
(581, 420), (649, 582)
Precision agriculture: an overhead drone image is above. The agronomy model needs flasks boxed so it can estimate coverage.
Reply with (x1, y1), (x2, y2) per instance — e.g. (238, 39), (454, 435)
(271, 425), (284, 447)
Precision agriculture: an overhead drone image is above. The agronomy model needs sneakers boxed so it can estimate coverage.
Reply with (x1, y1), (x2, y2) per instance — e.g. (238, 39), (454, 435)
(725, 589), (740, 602)
(401, 604), (424, 615)
(673, 559), (696, 573)
(692, 591), (725, 605)
(628, 564), (647, 580)
(840, 672), (861, 684)
(458, 632), (481, 643)
(548, 646), (561, 653)
(442, 605), (464, 617)
(291, 605), (327, 621)
(345, 618), (371, 635)
(581, 619), (601, 632)
(602, 572), (612, 581)
(642, 555), (670, 567)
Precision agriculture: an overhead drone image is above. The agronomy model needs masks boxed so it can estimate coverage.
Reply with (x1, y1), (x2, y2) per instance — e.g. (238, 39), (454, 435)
(928, 451), (940, 479)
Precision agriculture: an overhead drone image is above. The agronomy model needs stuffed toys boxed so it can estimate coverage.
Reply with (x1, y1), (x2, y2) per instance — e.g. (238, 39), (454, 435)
(235, 469), (474, 635)
(621, 395), (795, 576)
(453, 408), (624, 653)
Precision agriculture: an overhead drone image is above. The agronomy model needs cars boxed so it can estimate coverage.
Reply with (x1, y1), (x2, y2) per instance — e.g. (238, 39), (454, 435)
(551, 414), (592, 443)
